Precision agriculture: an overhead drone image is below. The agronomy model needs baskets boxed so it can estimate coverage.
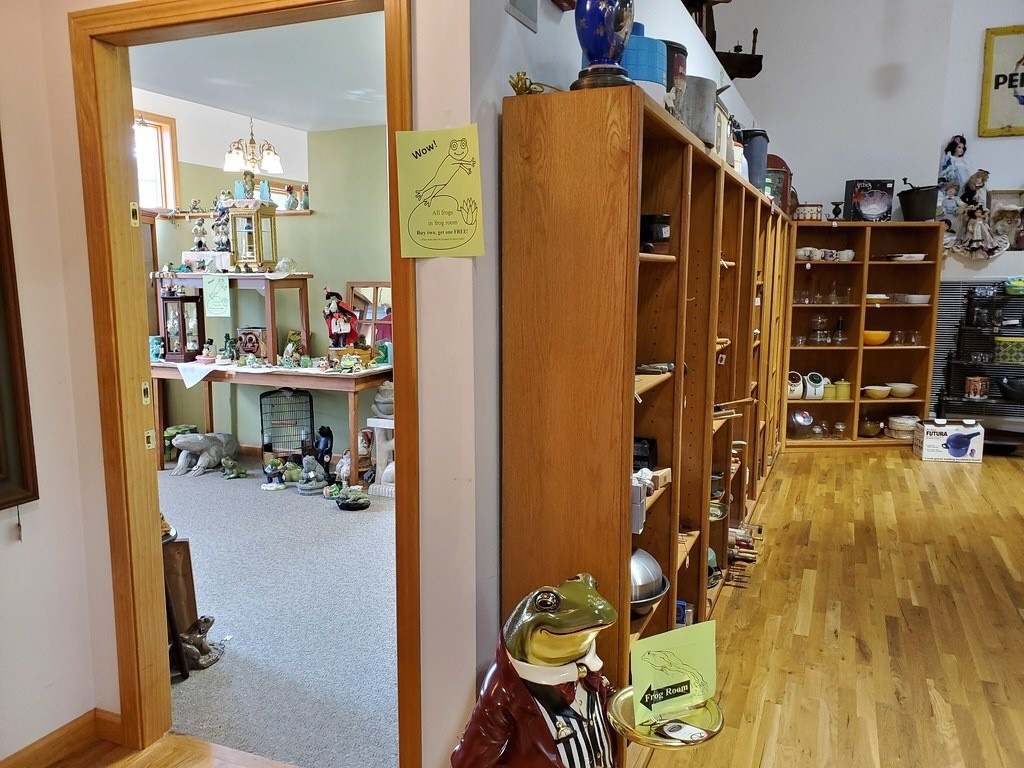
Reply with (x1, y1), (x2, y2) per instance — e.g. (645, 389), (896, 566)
(1006, 285), (1024, 295)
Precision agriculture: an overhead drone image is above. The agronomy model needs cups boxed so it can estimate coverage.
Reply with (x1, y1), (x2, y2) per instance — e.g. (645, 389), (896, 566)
(640, 214), (671, 253)
(965, 377), (989, 400)
(823, 379), (851, 401)
(796, 247), (855, 261)
(976, 286), (996, 297)
(971, 352), (992, 363)
(893, 330), (922, 345)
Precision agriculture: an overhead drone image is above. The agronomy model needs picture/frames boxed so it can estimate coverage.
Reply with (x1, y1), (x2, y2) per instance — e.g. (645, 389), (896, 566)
(978, 25), (1024, 137)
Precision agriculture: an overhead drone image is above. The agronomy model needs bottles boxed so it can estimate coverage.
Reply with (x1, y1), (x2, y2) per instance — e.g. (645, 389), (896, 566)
(793, 281), (841, 304)
(832, 315), (848, 346)
(972, 308), (1002, 327)
(809, 421), (847, 439)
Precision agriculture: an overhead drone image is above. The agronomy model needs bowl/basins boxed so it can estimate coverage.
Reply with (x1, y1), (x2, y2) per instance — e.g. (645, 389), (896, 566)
(855, 190), (891, 219)
(864, 331), (891, 344)
(866, 292), (932, 303)
(790, 316), (831, 347)
(630, 573), (670, 609)
(859, 383), (918, 437)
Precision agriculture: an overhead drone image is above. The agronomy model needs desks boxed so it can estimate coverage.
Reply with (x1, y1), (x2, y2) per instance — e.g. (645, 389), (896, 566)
(222, 199), (278, 272)
(367, 417), (394, 498)
(149, 270), (314, 365)
(149, 362), (393, 486)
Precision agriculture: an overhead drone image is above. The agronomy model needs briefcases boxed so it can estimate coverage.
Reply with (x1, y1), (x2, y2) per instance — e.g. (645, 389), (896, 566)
(993, 337), (1024, 365)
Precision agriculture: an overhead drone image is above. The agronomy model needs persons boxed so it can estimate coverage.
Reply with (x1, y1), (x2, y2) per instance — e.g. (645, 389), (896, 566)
(375, 308), (392, 342)
(937, 219), (956, 258)
(323, 287), (359, 348)
(937, 135), (969, 197)
(960, 169), (990, 207)
(936, 182), (969, 236)
(358, 428), (374, 482)
(952, 202), (1010, 260)
(335, 449), (351, 482)
(993, 204), (1024, 250)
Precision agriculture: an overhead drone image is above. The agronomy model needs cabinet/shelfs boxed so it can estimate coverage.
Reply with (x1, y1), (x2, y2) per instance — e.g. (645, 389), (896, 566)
(502, 86), (944, 767)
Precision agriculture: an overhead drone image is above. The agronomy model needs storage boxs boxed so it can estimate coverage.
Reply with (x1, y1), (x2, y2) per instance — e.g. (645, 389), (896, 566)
(843, 179), (895, 221)
(911, 419), (987, 464)
(738, 128), (770, 195)
(330, 347), (371, 365)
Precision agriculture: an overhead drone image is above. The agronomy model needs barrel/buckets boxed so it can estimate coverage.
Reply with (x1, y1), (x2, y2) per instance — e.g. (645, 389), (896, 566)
(897, 186), (942, 221)
(582, 22), (716, 149)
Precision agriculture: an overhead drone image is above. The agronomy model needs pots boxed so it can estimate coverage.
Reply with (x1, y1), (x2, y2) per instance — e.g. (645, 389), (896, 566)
(942, 432), (980, 457)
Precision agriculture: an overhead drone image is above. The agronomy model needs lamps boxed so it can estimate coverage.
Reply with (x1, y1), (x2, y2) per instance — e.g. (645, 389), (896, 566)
(223, 117), (283, 175)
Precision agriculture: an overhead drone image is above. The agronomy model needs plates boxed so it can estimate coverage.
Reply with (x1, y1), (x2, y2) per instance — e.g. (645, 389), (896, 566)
(888, 254), (928, 260)
